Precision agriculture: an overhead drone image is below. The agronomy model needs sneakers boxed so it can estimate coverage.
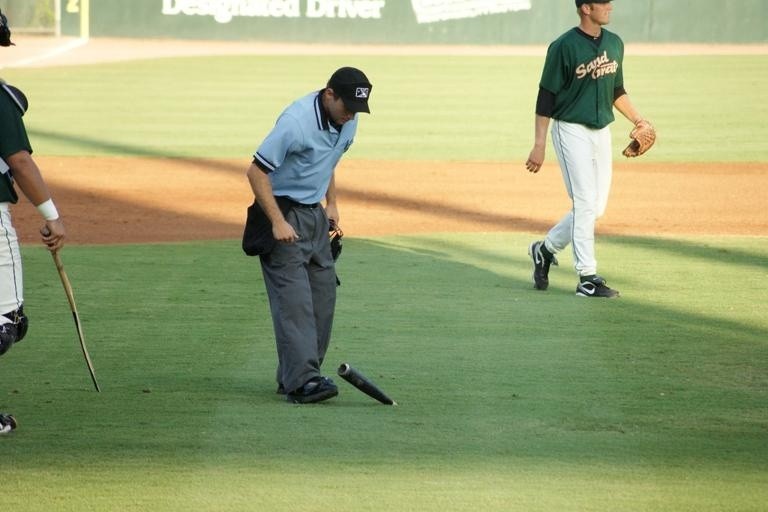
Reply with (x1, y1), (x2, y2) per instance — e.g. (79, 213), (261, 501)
(528, 241), (552, 291)
(0, 413), (17, 433)
(275, 377), (338, 405)
(575, 279), (619, 296)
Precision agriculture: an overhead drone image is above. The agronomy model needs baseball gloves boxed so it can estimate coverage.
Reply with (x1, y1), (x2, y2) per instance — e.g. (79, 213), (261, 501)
(622, 117), (655, 157)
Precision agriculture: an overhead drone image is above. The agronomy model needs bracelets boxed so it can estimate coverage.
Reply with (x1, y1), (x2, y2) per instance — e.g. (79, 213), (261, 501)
(36, 199), (59, 223)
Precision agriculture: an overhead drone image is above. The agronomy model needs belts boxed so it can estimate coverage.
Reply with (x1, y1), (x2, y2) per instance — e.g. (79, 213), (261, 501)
(297, 203), (318, 208)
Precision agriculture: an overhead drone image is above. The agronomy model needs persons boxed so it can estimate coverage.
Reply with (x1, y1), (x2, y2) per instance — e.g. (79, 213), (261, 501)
(246, 67), (372, 405)
(522, 0), (656, 296)
(1, 10), (71, 438)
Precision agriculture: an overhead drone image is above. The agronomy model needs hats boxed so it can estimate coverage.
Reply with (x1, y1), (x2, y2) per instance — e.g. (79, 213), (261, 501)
(327, 66), (370, 113)
